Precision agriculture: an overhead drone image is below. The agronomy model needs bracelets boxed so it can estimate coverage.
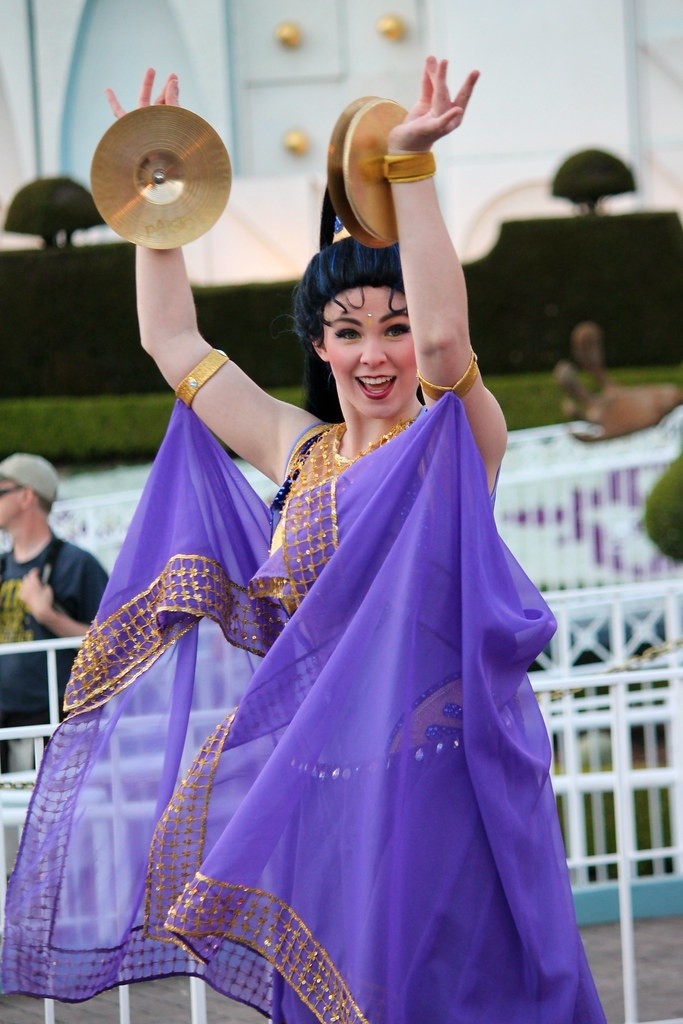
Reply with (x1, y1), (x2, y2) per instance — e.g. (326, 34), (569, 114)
(383, 150), (437, 183)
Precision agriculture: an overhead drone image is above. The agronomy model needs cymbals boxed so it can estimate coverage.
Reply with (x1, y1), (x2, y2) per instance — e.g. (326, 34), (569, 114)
(324, 95), (410, 251)
(90, 104), (231, 251)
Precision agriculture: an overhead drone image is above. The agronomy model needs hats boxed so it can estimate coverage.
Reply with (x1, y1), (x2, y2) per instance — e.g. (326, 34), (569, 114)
(0, 453), (58, 502)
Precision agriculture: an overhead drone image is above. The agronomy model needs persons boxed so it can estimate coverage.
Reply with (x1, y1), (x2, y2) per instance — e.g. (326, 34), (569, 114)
(100, 55), (509, 1024)
(0, 453), (109, 772)
